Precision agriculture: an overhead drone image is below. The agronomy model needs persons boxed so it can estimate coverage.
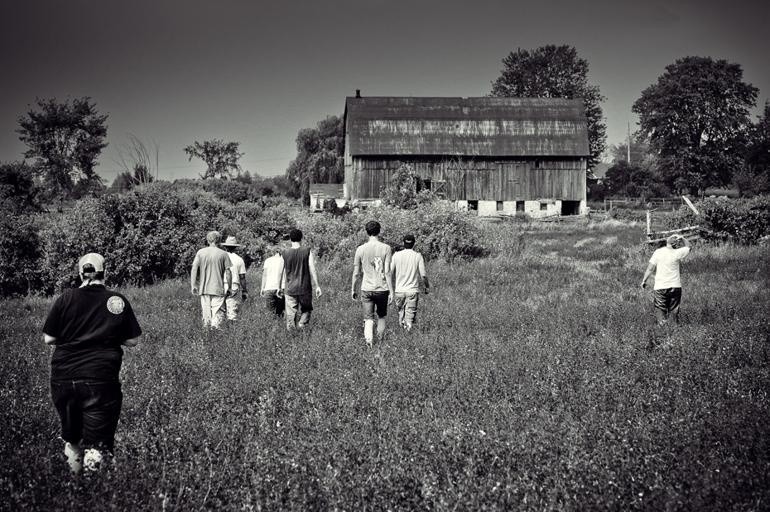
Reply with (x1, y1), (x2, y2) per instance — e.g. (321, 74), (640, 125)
(639, 236), (693, 323)
(389, 234), (428, 332)
(351, 221), (393, 347)
(219, 235), (248, 323)
(259, 247), (286, 319)
(42, 251), (143, 472)
(189, 230), (232, 329)
(275, 227), (323, 329)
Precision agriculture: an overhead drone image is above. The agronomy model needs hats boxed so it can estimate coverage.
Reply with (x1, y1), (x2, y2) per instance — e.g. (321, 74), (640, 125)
(403, 235), (415, 243)
(79, 253), (105, 277)
(220, 235), (241, 247)
